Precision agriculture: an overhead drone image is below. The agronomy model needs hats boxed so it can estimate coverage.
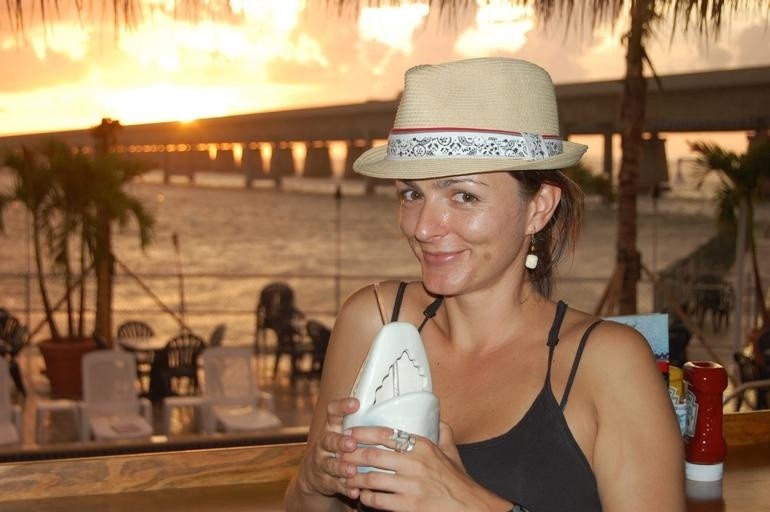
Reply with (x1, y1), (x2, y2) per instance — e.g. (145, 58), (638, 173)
(352, 57), (588, 181)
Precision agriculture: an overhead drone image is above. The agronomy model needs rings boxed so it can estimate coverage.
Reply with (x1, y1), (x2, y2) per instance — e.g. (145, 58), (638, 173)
(390, 429), (416, 454)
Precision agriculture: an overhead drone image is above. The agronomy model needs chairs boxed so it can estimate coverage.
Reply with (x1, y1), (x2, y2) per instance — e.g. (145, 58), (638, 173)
(0, 306), (282, 449)
(254, 283), (333, 379)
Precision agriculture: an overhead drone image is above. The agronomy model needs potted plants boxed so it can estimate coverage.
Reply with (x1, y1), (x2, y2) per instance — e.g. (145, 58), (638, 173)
(1, 119), (154, 399)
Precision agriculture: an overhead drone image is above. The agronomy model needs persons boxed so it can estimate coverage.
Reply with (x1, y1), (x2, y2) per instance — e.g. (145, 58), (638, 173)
(267, 287), (306, 387)
(281, 57), (688, 511)
